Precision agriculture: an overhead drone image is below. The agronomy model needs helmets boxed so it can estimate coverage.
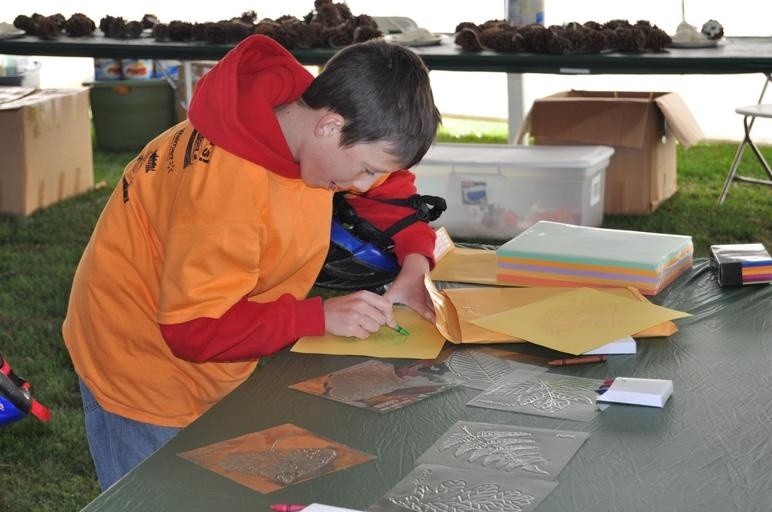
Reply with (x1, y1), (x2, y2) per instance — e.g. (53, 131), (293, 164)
(310, 188), (405, 294)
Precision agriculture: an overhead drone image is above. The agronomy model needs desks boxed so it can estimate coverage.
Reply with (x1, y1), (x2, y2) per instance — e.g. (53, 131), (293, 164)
(0, 16), (417, 120)
(381, 32), (772, 146)
(75, 241), (772, 512)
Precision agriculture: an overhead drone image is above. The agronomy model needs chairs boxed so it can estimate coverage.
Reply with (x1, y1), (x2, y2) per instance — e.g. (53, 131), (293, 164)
(716, 71), (772, 204)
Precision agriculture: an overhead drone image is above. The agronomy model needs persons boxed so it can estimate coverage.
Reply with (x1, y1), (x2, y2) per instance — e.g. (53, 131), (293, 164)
(61, 35), (442, 493)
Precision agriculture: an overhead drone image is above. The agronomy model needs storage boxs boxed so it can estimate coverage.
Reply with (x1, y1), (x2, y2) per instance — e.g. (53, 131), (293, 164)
(81, 79), (177, 153)
(509, 89), (705, 216)
(407, 142), (615, 241)
(0, 85), (95, 216)
(0, 59), (41, 89)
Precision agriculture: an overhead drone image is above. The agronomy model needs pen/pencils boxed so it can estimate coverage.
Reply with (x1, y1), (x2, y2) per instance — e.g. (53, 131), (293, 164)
(548, 355), (608, 366)
(269, 504), (306, 512)
(594, 376), (616, 395)
(387, 323), (411, 337)
